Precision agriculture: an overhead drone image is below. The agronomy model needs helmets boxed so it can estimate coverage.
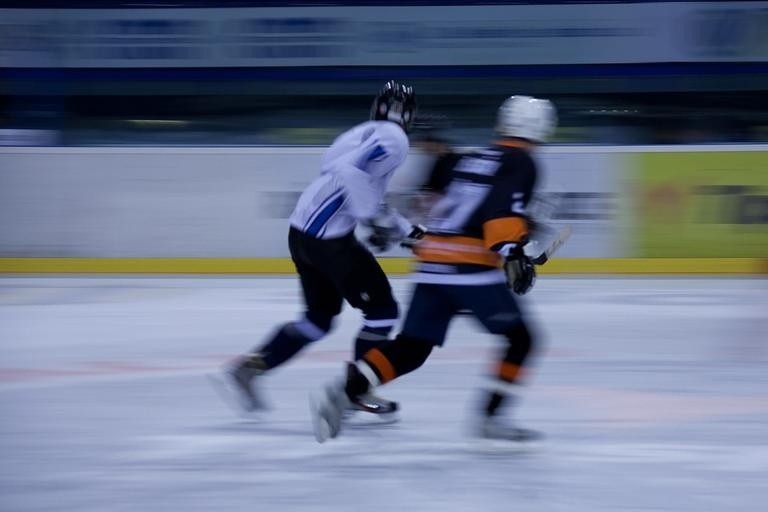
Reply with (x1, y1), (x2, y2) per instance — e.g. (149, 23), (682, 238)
(495, 92), (558, 146)
(370, 78), (418, 131)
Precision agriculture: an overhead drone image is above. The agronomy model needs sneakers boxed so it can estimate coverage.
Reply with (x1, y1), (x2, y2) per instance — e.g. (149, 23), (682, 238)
(228, 347), (265, 414)
(468, 414), (542, 442)
(319, 374), (401, 441)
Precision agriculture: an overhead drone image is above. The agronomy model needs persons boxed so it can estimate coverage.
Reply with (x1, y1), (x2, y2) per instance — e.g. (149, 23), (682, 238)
(316, 93), (560, 439)
(223, 79), (422, 415)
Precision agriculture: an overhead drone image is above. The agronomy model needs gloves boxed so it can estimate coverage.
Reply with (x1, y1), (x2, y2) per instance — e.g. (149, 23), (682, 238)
(366, 218), (427, 255)
(505, 245), (536, 296)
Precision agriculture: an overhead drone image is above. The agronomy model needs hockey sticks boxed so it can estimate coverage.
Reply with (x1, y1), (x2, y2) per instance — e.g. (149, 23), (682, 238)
(402, 224), (572, 266)
